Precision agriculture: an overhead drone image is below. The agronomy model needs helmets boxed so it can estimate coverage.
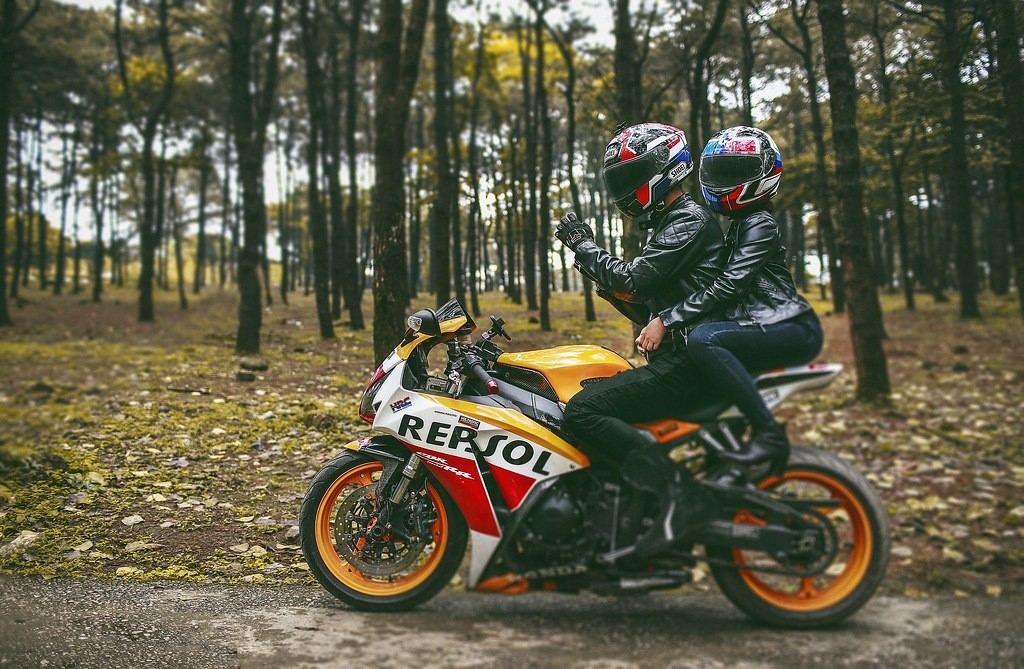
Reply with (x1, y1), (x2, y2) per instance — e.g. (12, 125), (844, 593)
(699, 126), (783, 218)
(602, 121), (695, 220)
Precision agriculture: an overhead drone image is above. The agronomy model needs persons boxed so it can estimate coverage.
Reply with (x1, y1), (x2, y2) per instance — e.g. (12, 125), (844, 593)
(555, 122), (732, 557)
(634, 126), (824, 480)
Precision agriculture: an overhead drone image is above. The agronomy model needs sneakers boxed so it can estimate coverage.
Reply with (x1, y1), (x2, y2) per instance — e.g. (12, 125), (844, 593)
(632, 480), (720, 560)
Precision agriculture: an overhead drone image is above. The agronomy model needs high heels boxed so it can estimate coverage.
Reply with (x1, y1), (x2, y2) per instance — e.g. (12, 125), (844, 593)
(713, 422), (791, 478)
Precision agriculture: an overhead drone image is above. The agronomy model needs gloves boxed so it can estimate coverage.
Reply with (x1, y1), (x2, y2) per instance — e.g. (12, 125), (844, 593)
(554, 213), (595, 255)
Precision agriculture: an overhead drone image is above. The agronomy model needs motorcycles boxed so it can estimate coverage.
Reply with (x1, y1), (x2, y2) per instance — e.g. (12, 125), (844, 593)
(299, 298), (890, 629)
